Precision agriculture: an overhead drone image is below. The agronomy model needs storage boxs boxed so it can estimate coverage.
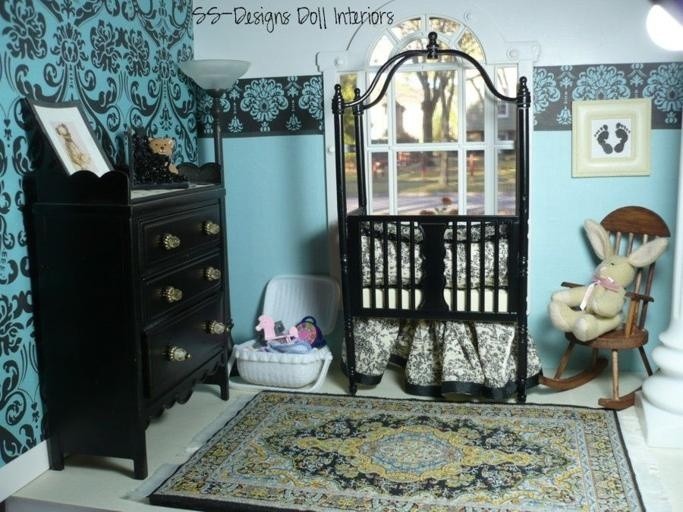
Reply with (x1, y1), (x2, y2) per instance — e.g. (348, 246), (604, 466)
(226, 274), (341, 392)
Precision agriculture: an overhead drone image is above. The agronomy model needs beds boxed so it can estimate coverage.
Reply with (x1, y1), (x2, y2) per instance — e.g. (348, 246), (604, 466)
(331, 31), (544, 403)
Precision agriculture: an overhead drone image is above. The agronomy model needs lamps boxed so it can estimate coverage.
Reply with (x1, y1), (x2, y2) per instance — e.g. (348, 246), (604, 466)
(646, 0), (683, 51)
(177, 59), (251, 377)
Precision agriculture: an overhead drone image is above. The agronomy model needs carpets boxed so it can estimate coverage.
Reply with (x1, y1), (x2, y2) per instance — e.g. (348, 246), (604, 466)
(147, 391), (646, 512)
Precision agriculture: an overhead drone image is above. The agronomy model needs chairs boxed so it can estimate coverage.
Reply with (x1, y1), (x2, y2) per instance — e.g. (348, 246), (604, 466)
(539, 206), (671, 409)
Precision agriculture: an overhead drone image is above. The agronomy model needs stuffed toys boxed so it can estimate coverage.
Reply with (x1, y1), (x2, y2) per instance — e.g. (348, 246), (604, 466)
(148, 135), (180, 177)
(545, 217), (669, 342)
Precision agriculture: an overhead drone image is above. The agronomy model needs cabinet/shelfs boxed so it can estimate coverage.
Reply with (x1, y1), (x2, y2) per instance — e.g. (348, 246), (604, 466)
(22, 162), (231, 480)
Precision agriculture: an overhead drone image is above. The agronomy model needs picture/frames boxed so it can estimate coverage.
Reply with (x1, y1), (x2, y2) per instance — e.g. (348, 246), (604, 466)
(572, 99), (651, 177)
(25, 97), (115, 177)
